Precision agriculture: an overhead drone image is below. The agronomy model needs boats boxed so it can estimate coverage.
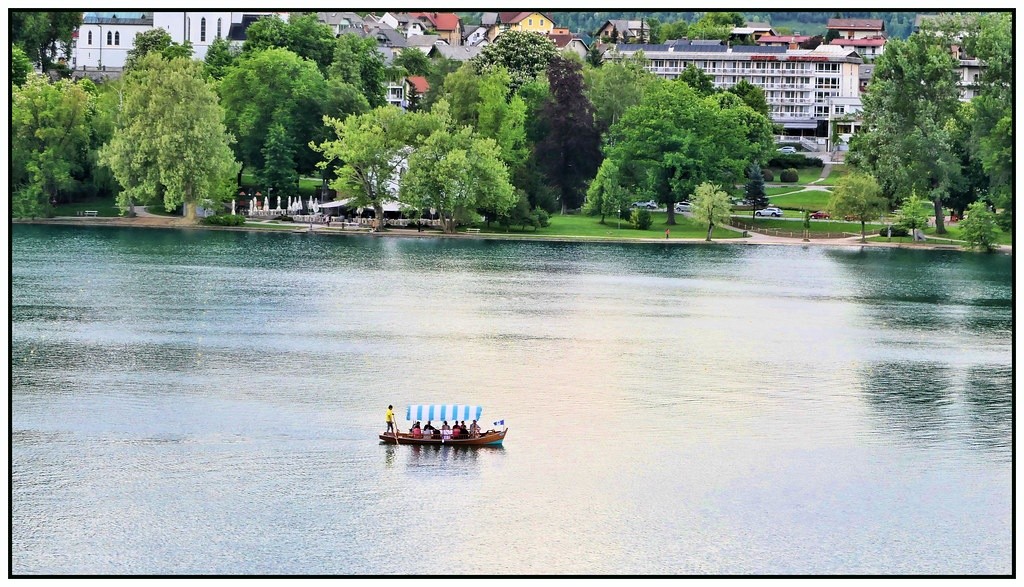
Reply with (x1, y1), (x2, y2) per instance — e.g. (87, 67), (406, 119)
(379, 404), (509, 446)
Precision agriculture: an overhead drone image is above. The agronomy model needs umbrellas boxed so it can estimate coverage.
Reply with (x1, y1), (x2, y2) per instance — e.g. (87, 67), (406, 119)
(231, 199), (235, 216)
(363, 198), (418, 212)
(249, 195), (319, 216)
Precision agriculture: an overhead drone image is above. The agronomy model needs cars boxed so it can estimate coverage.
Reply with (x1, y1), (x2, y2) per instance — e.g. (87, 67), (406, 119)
(776, 146), (797, 154)
(843, 213), (879, 222)
(879, 225), (910, 236)
(676, 202), (697, 212)
(809, 211), (831, 220)
(631, 199), (656, 208)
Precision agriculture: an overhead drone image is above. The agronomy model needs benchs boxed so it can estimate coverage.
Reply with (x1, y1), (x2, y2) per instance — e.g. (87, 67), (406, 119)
(396, 432), (471, 440)
(84, 210), (98, 218)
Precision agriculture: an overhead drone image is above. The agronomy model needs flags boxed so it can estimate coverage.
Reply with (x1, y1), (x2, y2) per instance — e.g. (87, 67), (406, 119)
(494, 420), (504, 426)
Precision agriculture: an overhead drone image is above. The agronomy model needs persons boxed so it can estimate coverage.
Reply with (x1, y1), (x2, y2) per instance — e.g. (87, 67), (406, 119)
(665, 229), (670, 238)
(409, 421), (421, 436)
(442, 421), (450, 430)
(386, 405), (394, 433)
(453, 420), (481, 437)
(424, 421), (436, 434)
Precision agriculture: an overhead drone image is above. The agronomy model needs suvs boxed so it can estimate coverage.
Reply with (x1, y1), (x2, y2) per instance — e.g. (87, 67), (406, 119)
(755, 208), (783, 217)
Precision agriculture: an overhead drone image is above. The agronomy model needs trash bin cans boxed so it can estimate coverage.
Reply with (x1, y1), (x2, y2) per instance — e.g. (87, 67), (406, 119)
(950, 215), (958, 221)
(743, 231), (747, 237)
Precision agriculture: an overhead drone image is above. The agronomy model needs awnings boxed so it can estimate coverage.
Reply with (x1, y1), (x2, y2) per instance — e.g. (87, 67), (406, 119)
(319, 197), (358, 216)
